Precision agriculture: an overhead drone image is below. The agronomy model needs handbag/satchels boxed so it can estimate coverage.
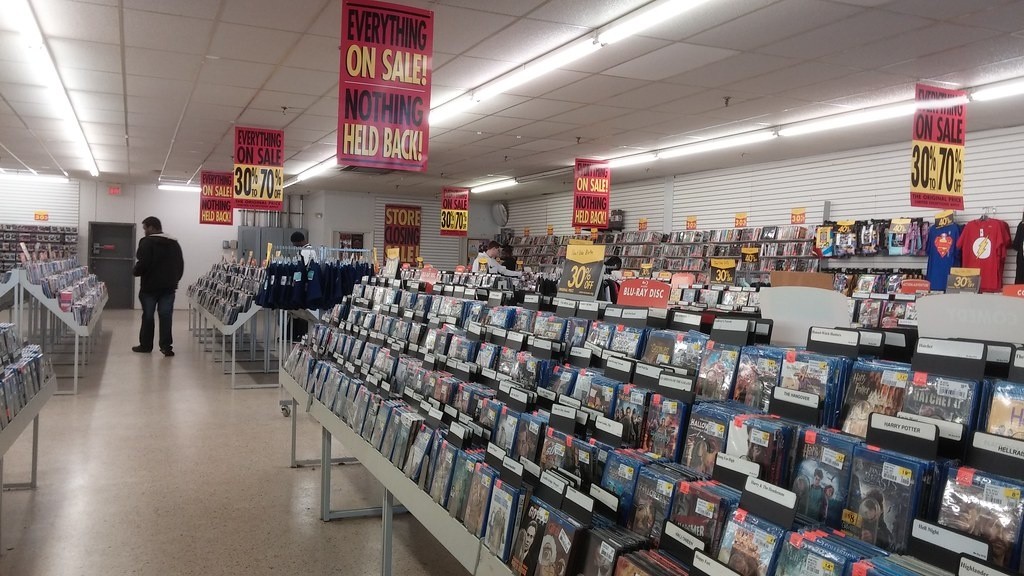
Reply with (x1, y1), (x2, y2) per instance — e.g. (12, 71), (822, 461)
(132, 260), (145, 276)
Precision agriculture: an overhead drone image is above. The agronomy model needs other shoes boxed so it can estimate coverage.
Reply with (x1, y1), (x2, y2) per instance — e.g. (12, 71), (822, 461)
(131, 344), (152, 354)
(160, 348), (174, 356)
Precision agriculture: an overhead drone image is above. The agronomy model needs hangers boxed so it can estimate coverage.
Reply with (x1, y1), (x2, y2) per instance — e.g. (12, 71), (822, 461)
(271, 245), (372, 265)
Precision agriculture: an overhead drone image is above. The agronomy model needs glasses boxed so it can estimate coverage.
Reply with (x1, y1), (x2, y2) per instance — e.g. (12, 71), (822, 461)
(527, 533), (534, 538)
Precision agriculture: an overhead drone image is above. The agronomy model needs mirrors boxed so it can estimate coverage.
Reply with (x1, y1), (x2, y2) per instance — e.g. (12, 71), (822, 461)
(491, 202), (508, 226)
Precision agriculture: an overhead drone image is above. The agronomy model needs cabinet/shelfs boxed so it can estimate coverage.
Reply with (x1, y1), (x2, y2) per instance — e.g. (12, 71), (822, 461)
(0, 224), (79, 281)
(186, 262), (284, 389)
(279, 223), (1024, 576)
(0, 268), (19, 340)
(19, 257), (110, 395)
(0, 321), (58, 530)
(242, 262), (318, 372)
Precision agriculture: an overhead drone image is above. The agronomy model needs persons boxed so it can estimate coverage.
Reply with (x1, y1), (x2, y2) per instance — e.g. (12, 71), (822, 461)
(277, 231), (311, 343)
(501, 244), (517, 271)
(132, 217), (184, 356)
(615, 407), (642, 444)
(793, 468), (835, 523)
(472, 240), (527, 278)
(844, 369), (903, 438)
(645, 418), (675, 457)
(512, 521), (538, 576)
